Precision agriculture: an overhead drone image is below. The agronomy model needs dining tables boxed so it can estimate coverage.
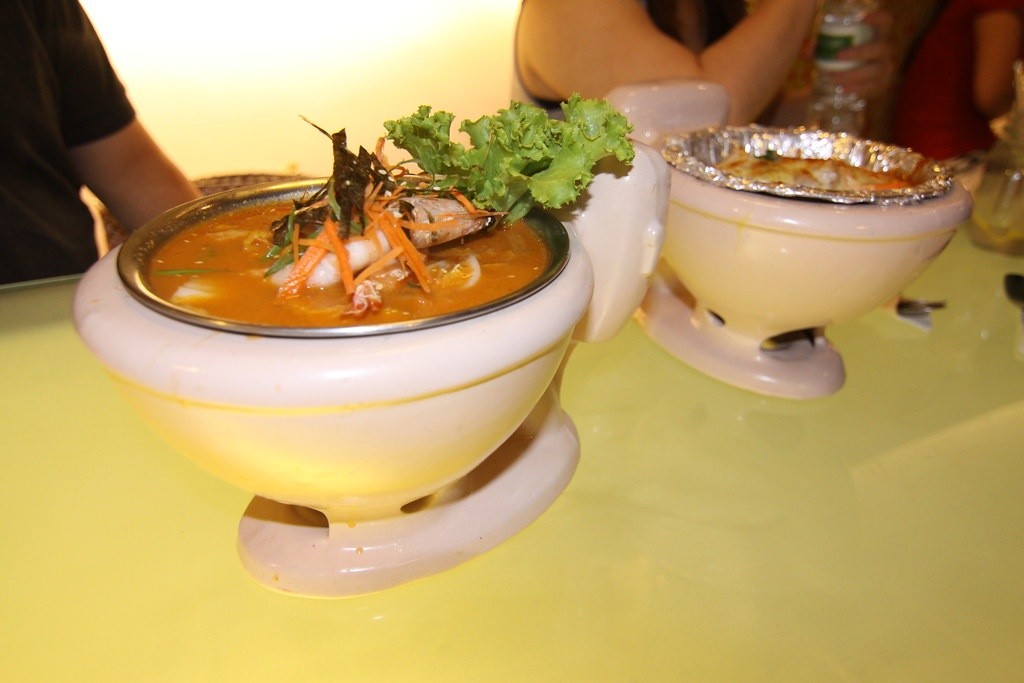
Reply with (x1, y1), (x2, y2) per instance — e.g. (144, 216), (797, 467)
(0, 220), (1024, 683)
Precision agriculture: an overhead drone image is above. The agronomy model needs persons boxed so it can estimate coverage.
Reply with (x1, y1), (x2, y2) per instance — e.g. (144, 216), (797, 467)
(511, 0), (1024, 160)
(0, 0), (205, 285)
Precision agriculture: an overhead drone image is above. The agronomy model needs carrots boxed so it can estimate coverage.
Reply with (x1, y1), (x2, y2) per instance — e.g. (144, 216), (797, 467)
(275, 136), (476, 298)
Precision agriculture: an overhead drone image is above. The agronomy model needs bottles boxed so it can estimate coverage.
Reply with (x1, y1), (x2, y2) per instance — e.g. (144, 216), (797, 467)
(971, 64), (1024, 248)
(808, 0), (879, 141)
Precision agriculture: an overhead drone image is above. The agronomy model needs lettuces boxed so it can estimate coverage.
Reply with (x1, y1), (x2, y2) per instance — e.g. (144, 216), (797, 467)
(385, 91), (637, 227)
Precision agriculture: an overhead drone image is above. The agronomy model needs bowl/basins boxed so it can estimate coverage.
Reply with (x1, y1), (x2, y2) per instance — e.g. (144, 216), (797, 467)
(605, 81), (970, 402)
(75, 137), (672, 593)
